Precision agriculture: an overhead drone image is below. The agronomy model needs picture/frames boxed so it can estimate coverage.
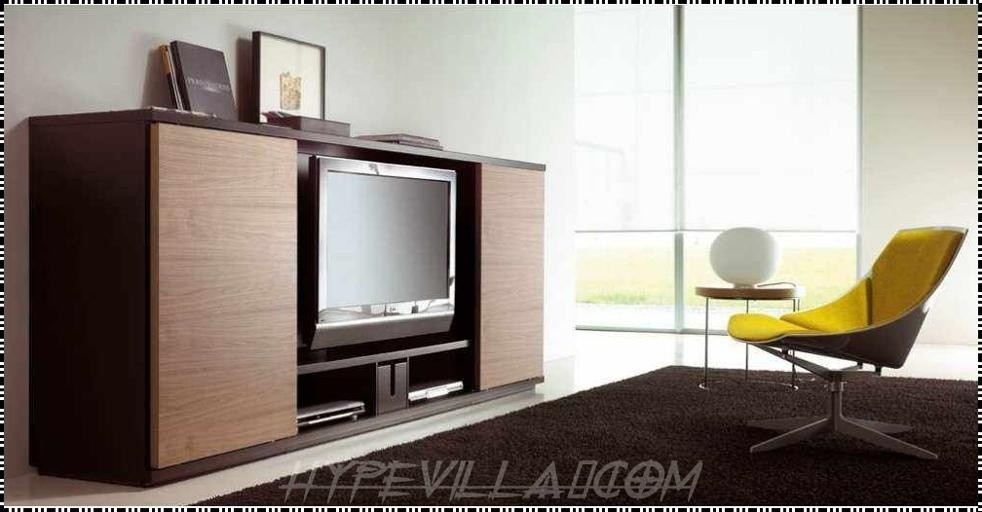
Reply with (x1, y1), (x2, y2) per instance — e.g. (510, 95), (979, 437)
(252, 31), (325, 123)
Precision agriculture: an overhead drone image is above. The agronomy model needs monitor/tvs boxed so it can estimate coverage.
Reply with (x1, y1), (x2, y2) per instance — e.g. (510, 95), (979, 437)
(305, 154), (457, 351)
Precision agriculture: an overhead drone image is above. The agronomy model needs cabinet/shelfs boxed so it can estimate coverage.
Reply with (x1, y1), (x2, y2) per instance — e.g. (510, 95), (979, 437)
(28, 108), (546, 488)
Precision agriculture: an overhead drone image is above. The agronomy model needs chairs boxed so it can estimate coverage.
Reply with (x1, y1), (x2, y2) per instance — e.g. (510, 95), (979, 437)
(727, 226), (968, 459)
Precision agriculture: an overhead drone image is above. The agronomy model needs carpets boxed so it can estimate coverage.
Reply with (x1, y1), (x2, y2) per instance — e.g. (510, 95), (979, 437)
(188, 366), (978, 507)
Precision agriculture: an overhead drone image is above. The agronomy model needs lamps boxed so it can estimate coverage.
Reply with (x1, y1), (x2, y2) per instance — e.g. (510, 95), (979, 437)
(709, 227), (779, 289)
